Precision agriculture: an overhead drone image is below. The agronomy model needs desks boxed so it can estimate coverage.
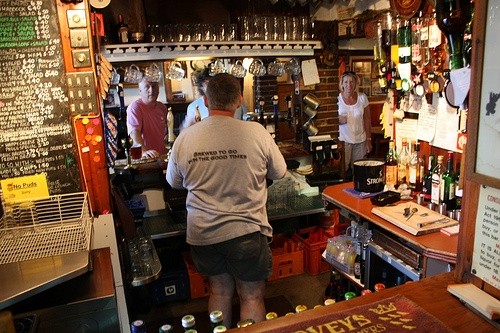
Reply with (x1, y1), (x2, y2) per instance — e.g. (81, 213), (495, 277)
(321, 179), (460, 290)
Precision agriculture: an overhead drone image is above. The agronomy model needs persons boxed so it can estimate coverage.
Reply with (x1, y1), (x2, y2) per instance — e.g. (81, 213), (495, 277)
(126, 76), (173, 158)
(338, 71), (375, 184)
(165, 73), (286, 330)
(179, 67), (243, 132)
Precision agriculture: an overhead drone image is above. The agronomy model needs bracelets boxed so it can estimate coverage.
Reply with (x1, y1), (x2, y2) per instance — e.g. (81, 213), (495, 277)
(366, 138), (371, 140)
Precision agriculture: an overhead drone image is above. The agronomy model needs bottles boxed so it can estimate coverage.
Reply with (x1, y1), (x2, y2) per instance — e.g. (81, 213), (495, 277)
(430, 155), (446, 205)
(386, 137), (426, 192)
(354, 241), (362, 279)
(422, 155), (436, 194)
(440, 150), (455, 210)
(360, 243), (369, 284)
(130, 270), (417, 333)
(375, 0), (474, 93)
(104, 14), (130, 44)
(455, 174), (463, 210)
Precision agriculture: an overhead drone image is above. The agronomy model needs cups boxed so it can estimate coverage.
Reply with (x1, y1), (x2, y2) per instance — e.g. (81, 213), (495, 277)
(266, 176), (301, 210)
(417, 193), (461, 220)
(131, 144), (143, 162)
(342, 112), (348, 124)
(110, 61), (185, 85)
(127, 235), (154, 278)
(302, 92), (321, 136)
(209, 57), (301, 79)
(132, 14), (321, 43)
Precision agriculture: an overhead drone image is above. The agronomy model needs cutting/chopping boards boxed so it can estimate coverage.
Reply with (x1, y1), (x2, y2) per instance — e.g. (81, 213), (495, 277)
(371, 201), (459, 235)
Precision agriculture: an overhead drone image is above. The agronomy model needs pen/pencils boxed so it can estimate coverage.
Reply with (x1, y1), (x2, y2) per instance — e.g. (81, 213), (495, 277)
(406, 208), (417, 220)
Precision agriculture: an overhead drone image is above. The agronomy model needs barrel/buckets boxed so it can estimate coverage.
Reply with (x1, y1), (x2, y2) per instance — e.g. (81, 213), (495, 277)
(352, 159), (386, 193)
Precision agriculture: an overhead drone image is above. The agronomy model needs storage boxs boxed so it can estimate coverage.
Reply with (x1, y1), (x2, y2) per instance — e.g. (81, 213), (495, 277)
(181, 223), (351, 299)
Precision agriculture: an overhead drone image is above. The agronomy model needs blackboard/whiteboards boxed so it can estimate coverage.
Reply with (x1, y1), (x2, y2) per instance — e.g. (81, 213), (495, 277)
(0, 1), (83, 231)
